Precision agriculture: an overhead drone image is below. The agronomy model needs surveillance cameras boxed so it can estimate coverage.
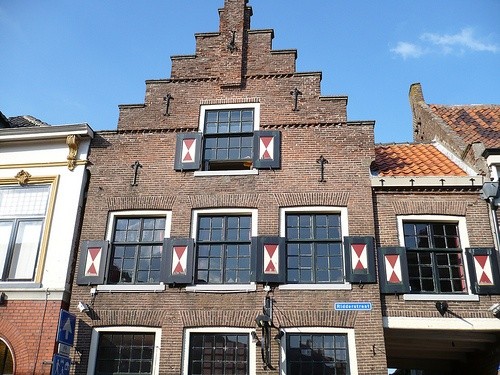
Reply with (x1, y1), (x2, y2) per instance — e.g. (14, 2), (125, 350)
(76, 301), (90, 313)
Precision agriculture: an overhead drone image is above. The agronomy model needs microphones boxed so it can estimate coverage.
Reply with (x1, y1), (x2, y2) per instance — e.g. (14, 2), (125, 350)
(487, 303), (500, 316)
